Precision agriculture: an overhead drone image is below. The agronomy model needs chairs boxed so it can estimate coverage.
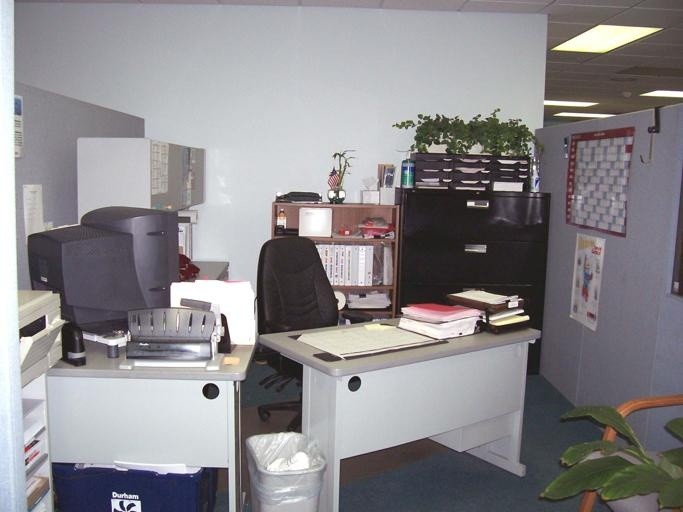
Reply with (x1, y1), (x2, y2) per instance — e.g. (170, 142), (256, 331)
(256, 236), (373, 432)
(577, 394), (683, 512)
(169, 280), (256, 346)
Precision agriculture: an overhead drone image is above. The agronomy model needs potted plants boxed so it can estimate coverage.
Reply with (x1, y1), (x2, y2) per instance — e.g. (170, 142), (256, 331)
(392, 107), (544, 158)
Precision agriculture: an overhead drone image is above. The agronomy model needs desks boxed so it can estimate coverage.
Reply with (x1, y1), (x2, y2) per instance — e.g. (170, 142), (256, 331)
(259, 317), (541, 512)
(48, 343), (255, 512)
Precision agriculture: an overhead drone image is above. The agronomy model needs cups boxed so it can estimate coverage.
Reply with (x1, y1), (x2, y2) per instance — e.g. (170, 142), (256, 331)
(107, 344), (120, 359)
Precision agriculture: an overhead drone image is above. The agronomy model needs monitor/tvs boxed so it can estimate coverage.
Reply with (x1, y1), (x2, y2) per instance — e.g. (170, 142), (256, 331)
(27, 206), (180, 337)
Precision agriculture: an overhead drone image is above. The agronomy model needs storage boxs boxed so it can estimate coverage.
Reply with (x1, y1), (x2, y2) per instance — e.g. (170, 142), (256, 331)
(52, 463), (218, 512)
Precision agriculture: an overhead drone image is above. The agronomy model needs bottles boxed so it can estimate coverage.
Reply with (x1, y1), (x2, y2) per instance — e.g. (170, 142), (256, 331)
(531, 171), (540, 192)
(66, 324), (86, 366)
(277, 208), (286, 229)
(401, 156), (416, 189)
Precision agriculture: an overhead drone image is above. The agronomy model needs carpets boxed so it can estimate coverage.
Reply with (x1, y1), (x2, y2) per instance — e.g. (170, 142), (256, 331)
(240, 347), (622, 512)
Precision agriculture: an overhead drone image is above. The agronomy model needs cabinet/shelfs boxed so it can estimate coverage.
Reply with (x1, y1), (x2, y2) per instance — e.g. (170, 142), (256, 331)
(77, 137), (230, 282)
(271, 200), (400, 325)
(395, 188), (551, 375)
(19, 293), (63, 512)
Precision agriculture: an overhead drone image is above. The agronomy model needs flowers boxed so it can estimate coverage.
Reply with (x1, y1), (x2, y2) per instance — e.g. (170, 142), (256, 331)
(328, 150), (355, 195)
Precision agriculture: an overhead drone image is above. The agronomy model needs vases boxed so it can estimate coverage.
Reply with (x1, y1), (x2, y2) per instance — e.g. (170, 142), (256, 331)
(326, 186), (347, 204)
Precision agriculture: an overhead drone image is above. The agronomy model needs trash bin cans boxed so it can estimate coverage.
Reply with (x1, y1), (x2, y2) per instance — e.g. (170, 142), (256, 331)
(245, 432), (326, 512)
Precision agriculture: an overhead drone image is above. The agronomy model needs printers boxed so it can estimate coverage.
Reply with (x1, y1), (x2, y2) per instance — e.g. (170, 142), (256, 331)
(17, 289), (63, 388)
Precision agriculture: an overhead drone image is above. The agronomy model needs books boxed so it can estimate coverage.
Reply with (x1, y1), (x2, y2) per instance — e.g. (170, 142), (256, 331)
(25, 438), (41, 470)
(397, 289), (530, 341)
(177, 217), (192, 260)
(348, 291), (390, 309)
(314, 244), (373, 287)
